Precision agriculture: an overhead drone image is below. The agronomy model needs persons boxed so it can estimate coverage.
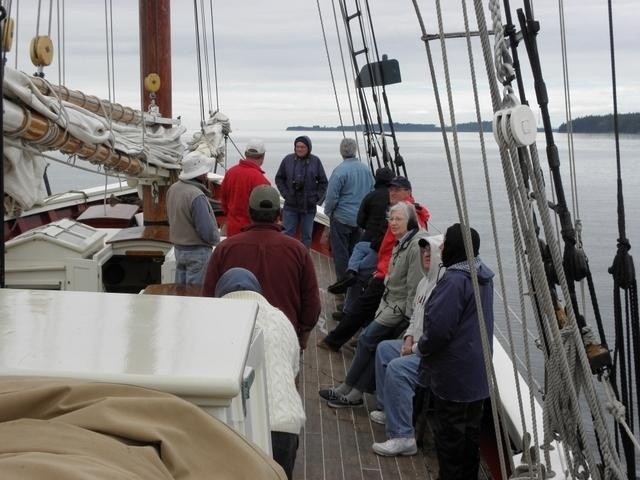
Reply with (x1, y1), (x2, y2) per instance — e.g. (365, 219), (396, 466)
(215, 266), (306, 480)
(165, 149), (221, 285)
(274, 136), (329, 250)
(219, 139), (271, 239)
(201, 182), (322, 389)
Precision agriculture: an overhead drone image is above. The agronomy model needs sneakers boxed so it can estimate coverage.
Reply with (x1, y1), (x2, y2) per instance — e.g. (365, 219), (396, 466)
(317, 336), (331, 351)
(331, 303), (345, 321)
(371, 437), (418, 458)
(368, 410), (386, 425)
(327, 269), (358, 294)
(318, 388), (343, 400)
(327, 394), (364, 408)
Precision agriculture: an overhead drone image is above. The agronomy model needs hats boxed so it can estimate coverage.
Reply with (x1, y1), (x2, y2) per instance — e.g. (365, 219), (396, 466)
(386, 176), (411, 190)
(177, 149), (217, 181)
(245, 139), (269, 155)
(373, 167), (396, 189)
(248, 184), (281, 213)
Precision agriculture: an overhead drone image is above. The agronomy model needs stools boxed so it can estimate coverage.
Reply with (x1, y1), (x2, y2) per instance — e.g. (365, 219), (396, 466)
(412, 383), (444, 448)
